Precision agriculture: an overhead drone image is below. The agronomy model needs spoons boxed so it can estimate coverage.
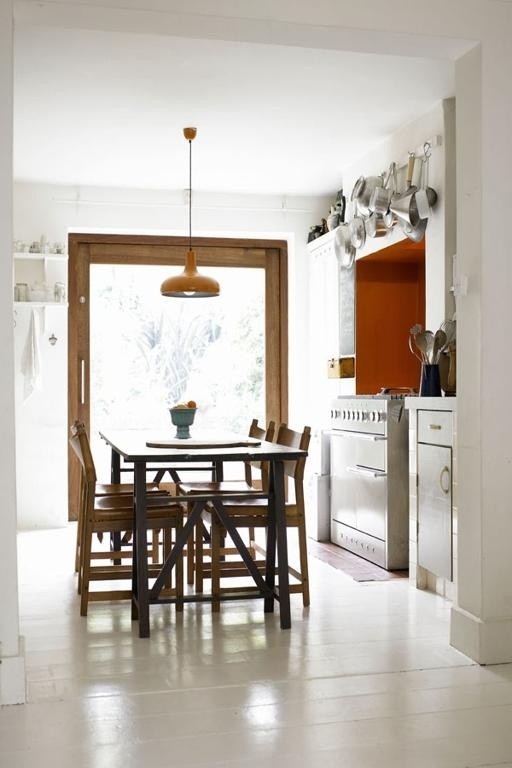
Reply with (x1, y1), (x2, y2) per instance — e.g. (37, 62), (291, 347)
(409, 332), (429, 365)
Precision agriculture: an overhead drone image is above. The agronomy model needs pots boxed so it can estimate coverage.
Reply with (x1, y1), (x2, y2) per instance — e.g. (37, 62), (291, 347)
(334, 155), (430, 269)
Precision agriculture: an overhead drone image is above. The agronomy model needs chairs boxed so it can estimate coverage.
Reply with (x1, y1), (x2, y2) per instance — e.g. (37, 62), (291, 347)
(177, 417), (310, 612)
(65, 419), (184, 616)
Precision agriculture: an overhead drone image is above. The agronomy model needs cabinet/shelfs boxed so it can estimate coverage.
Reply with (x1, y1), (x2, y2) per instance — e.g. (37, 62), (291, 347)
(13, 252), (69, 308)
(404, 397), (457, 604)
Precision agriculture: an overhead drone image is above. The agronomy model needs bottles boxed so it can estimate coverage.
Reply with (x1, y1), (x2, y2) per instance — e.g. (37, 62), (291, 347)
(15, 283), (29, 302)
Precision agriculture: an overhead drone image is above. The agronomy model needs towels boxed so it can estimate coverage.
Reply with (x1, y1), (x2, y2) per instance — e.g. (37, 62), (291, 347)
(20, 309), (43, 405)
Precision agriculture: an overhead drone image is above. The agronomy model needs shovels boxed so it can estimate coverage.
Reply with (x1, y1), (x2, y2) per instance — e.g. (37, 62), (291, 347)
(415, 142), (433, 221)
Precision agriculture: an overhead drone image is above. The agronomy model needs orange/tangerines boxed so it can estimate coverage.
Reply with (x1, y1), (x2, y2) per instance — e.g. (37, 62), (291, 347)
(188, 401), (196, 408)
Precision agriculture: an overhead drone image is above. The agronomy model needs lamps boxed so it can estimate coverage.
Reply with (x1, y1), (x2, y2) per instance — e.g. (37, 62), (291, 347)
(160, 127), (219, 299)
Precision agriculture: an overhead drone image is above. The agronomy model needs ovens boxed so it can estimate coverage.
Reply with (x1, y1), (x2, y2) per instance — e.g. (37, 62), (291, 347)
(330, 395), (409, 571)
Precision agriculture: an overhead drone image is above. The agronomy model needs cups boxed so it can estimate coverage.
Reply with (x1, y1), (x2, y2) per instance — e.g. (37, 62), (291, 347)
(421, 364), (442, 397)
